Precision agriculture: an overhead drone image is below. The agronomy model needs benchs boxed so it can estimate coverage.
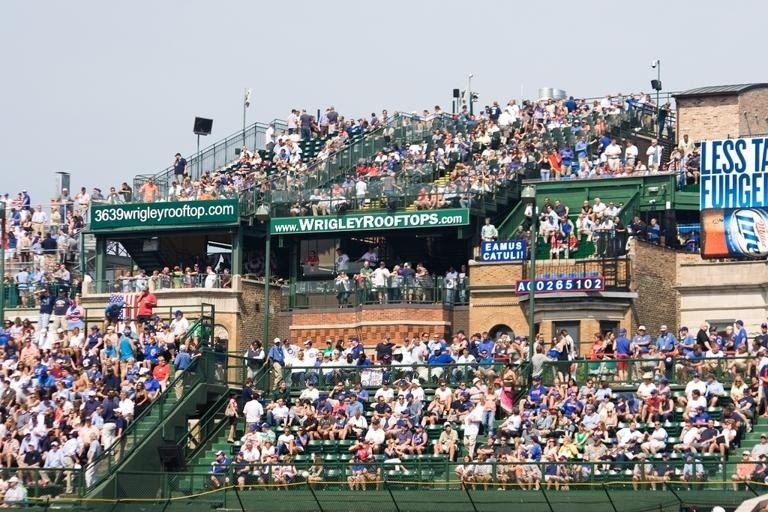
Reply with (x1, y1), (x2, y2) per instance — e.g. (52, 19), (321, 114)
(508, 206), (616, 261)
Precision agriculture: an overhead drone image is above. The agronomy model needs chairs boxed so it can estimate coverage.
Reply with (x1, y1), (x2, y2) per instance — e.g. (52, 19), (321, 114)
(229, 359), (760, 488)
(199, 106), (640, 209)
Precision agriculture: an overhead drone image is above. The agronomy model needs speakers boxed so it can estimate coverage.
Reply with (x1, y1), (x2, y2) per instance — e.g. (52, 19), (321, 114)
(651, 80), (658, 89)
(656, 80), (661, 90)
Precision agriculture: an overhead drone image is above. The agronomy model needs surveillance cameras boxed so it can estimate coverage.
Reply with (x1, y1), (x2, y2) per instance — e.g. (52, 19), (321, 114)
(245, 100), (250, 107)
(651, 61), (656, 68)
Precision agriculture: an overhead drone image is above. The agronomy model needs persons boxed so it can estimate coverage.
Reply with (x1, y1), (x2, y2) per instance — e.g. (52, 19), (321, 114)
(137, 177), (161, 203)
(454, 389), (758, 492)
(731, 433), (768, 491)
(335, 245), (467, 309)
(631, 215), (660, 242)
(112, 263), (232, 292)
(0, 310), (200, 508)
(167, 104), (390, 216)
(481, 218), (498, 242)
(243, 340), (266, 382)
(103, 286), (157, 333)
(210, 362), (517, 489)
(667, 134), (701, 190)
(518, 93), (671, 182)
(384, 93), (523, 211)
(89, 183), (133, 204)
(0, 185), (89, 346)
(305, 250), (319, 271)
(213, 336), (225, 385)
(268, 319), (768, 417)
(516, 196), (626, 259)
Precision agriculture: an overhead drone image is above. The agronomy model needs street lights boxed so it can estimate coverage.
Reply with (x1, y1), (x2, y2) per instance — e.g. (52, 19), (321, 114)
(253, 203), (272, 393)
(520, 184), (538, 391)
